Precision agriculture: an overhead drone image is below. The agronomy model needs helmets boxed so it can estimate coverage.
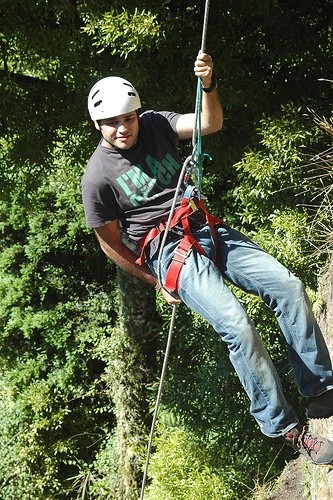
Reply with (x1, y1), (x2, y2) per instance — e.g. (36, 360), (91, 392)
(86, 75), (142, 131)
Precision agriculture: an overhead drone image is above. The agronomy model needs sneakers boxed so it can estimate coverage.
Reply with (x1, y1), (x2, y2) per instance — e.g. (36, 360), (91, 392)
(307, 389), (333, 419)
(279, 422), (333, 465)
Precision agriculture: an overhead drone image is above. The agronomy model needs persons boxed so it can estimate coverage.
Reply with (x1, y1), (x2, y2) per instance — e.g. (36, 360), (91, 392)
(81, 50), (332, 465)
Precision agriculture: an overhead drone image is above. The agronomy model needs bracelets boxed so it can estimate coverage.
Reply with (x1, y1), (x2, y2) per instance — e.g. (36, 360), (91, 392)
(196, 77), (219, 94)
